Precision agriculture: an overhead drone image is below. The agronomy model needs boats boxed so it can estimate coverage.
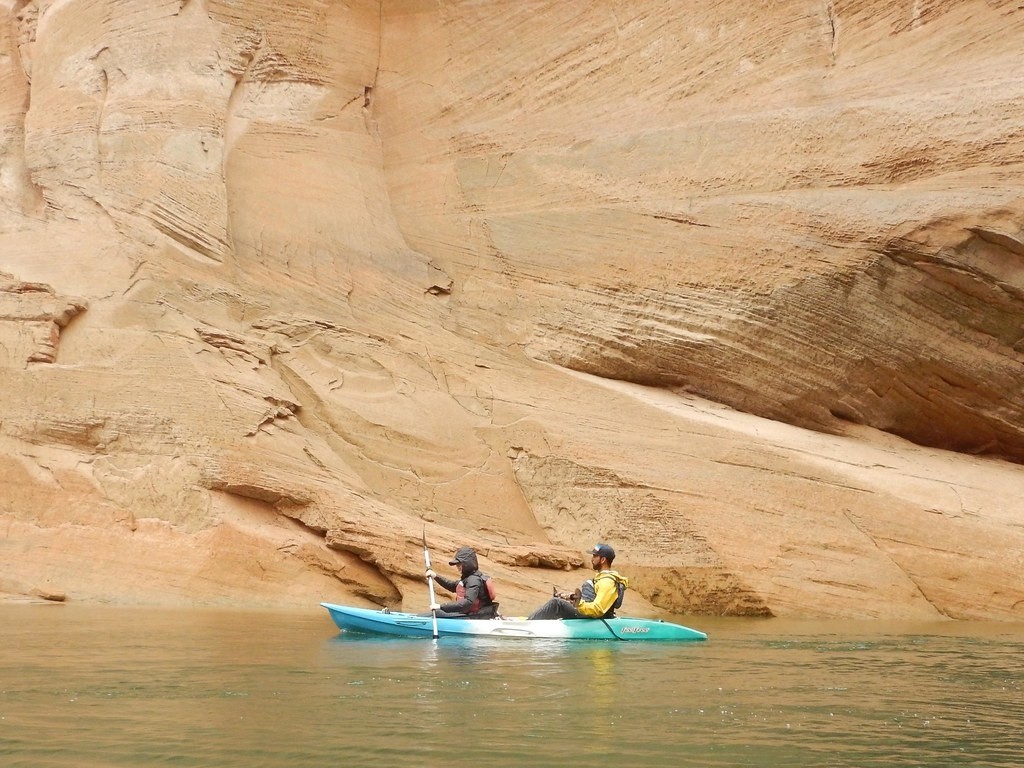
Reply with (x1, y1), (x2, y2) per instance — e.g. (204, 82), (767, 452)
(318, 604), (709, 642)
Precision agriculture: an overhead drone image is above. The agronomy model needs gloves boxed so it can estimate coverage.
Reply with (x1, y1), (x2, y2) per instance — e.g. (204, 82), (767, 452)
(555, 591), (564, 597)
(430, 603), (440, 612)
(561, 592), (575, 603)
(426, 570), (436, 579)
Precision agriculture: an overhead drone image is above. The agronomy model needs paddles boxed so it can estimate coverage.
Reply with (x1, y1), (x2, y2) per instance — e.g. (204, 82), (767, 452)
(553, 586), (582, 606)
(423, 523), (439, 640)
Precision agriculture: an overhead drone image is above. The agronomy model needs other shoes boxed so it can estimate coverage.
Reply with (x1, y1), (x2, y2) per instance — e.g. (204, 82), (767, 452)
(495, 613), (508, 621)
(382, 607), (390, 614)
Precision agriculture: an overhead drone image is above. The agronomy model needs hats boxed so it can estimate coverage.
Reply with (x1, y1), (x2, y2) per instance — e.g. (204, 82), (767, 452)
(586, 544), (615, 559)
(449, 558), (460, 565)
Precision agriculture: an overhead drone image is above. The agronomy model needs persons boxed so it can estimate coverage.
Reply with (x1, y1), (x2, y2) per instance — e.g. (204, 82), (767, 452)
(426, 547), (495, 619)
(520, 543), (629, 620)
